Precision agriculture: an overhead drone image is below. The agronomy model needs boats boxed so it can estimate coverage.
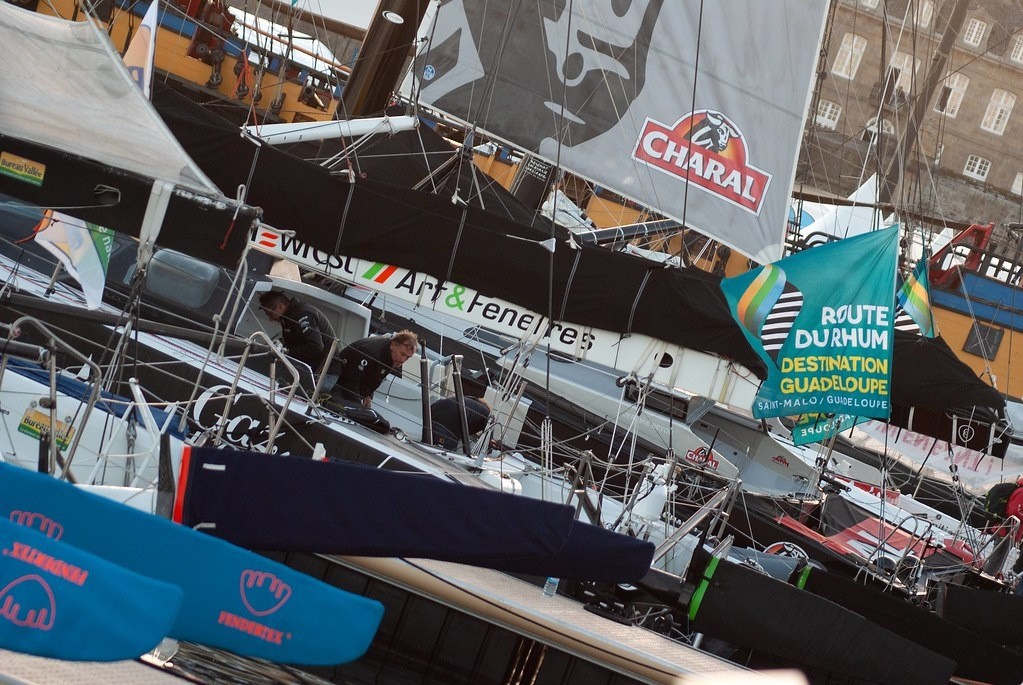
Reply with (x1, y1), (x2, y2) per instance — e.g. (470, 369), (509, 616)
(0, 0), (1023, 685)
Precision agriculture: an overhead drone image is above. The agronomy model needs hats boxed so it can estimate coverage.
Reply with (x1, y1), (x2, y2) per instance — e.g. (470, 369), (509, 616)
(258, 291), (286, 313)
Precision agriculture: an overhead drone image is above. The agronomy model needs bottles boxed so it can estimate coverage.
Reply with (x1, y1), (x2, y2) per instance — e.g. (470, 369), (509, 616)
(1005, 549), (1021, 576)
(543, 576), (560, 598)
(1002, 547), (1016, 573)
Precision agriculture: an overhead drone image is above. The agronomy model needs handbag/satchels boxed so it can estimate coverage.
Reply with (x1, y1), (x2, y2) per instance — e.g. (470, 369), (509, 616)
(343, 403), (390, 435)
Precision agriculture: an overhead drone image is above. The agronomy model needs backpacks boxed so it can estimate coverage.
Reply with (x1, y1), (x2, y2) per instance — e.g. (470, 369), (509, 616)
(984, 482), (1023, 532)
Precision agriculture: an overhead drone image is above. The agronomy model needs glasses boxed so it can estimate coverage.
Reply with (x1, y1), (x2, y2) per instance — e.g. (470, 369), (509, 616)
(396, 341), (419, 354)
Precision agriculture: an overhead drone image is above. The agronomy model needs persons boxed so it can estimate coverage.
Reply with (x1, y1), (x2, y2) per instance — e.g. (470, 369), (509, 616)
(328, 330), (417, 408)
(431, 395), (493, 455)
(262, 291), (340, 401)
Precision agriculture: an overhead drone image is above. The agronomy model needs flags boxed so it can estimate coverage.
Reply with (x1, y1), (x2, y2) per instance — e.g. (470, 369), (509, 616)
(720, 221), (899, 444)
(896, 253), (934, 338)
(34, 208), (115, 311)
